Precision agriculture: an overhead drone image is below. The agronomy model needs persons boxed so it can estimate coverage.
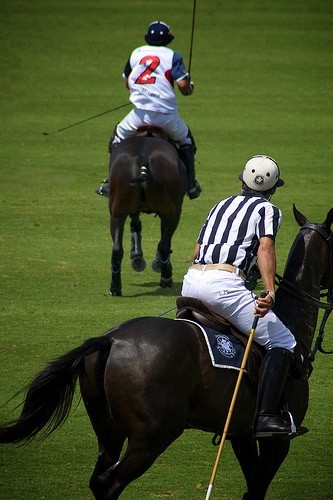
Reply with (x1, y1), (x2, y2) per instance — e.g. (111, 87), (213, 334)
(181, 154), (310, 438)
(94, 21), (201, 200)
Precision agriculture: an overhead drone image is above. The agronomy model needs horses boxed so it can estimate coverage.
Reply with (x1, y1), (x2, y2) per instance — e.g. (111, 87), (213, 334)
(1, 203), (333, 500)
(109, 122), (188, 297)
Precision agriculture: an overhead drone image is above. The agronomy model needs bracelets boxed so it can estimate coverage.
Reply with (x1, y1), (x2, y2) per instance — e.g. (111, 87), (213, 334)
(268, 291), (275, 303)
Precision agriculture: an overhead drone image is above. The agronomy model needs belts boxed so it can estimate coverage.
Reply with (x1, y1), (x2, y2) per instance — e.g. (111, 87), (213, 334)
(190, 264), (246, 281)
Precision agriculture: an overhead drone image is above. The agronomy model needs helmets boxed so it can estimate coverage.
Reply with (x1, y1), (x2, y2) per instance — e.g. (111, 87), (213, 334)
(239, 154), (284, 192)
(144, 21), (175, 45)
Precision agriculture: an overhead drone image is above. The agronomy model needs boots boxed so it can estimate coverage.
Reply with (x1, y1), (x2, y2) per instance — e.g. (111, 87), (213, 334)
(255, 346), (308, 438)
(180, 148), (202, 199)
(95, 183), (111, 197)
